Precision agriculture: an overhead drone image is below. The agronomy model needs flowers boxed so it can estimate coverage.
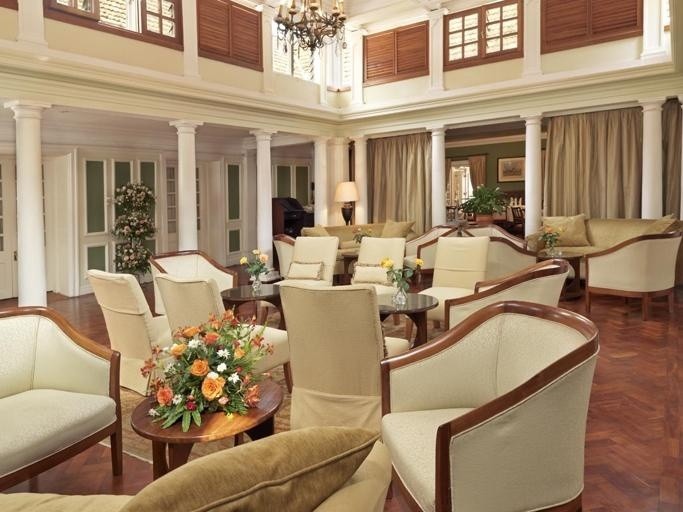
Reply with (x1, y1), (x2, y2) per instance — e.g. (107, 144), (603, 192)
(110, 179), (156, 276)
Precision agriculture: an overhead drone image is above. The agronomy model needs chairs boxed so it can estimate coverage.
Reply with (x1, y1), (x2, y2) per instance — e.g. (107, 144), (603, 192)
(507, 206), (525, 239)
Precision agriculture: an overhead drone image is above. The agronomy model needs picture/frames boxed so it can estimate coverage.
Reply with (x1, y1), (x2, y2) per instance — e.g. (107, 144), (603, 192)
(496, 155), (525, 183)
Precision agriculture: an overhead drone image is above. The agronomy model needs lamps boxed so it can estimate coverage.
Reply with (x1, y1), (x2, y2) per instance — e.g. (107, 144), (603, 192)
(334, 181), (360, 226)
(275, 1), (349, 55)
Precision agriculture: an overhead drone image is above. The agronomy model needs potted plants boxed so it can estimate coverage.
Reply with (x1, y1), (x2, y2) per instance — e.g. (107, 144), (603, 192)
(461, 182), (506, 225)
